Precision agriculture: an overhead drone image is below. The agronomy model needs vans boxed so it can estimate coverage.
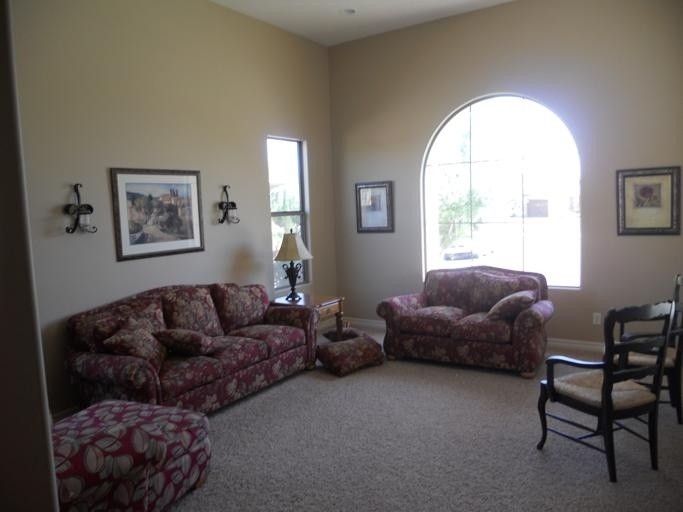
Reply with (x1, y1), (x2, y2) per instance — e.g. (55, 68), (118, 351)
(443, 223), (521, 271)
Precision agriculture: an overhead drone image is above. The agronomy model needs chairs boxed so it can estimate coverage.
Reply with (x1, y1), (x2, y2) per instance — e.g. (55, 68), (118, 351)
(598, 272), (682, 408)
(533, 297), (676, 483)
(609, 304), (683, 425)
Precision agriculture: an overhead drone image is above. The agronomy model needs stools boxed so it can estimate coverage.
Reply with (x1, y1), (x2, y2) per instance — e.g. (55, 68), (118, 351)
(52, 396), (214, 511)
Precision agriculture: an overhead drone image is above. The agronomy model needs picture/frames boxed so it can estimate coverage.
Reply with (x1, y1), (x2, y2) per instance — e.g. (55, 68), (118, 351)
(351, 179), (395, 235)
(613, 164), (683, 238)
(107, 164), (205, 263)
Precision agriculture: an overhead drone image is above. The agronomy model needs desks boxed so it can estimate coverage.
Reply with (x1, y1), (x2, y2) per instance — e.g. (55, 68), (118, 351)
(270, 291), (345, 345)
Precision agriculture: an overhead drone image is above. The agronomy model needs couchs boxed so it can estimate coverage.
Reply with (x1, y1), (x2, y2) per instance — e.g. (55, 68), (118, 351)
(62, 280), (323, 418)
(374, 264), (554, 380)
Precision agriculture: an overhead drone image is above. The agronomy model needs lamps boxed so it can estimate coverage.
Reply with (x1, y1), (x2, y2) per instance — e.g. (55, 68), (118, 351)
(60, 178), (98, 236)
(214, 182), (243, 226)
(272, 228), (316, 302)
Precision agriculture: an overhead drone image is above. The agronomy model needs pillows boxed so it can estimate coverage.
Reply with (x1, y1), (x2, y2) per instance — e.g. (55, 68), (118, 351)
(313, 333), (385, 379)
(149, 326), (226, 357)
(321, 324), (383, 350)
(483, 287), (538, 322)
(100, 313), (172, 376)
